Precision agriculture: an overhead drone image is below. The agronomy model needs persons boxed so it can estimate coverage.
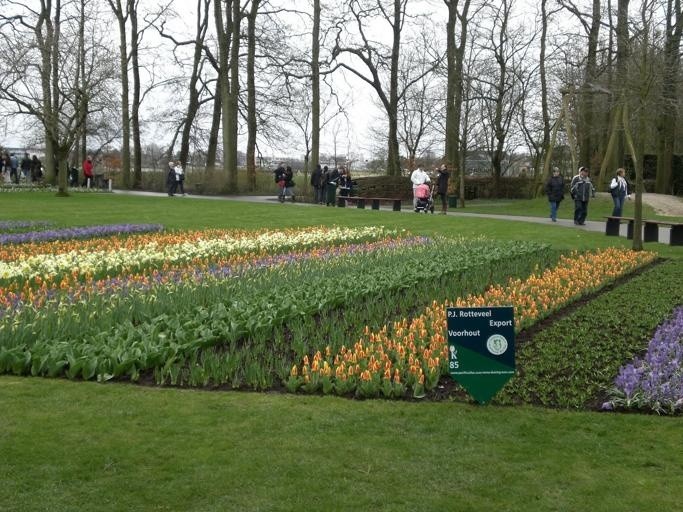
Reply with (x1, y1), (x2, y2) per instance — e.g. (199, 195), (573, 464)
(543, 166), (566, 222)
(165, 161), (176, 196)
(569, 166), (596, 226)
(173, 160), (186, 194)
(434, 164), (449, 215)
(270, 163), (353, 210)
(0, 151), (109, 192)
(609, 167), (632, 216)
(410, 163), (430, 210)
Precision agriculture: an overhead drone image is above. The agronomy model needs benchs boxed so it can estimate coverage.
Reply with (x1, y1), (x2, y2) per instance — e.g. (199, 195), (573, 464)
(601, 216), (683, 246)
(335, 196), (406, 211)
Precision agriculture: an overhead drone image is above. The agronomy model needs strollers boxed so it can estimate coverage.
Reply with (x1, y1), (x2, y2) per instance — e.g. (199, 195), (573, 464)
(414, 182), (435, 214)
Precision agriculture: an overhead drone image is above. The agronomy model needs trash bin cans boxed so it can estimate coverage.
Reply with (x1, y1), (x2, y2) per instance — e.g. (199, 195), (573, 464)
(447, 195), (457, 208)
(326, 182), (337, 207)
